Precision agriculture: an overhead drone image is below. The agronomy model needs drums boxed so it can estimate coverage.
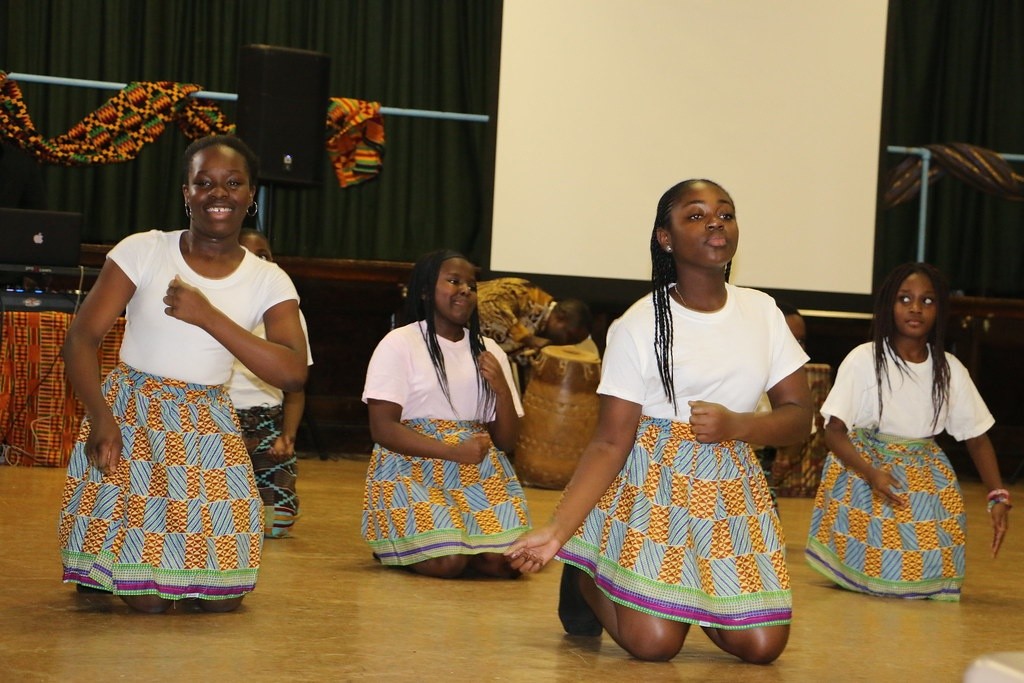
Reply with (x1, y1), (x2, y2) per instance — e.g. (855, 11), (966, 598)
(769, 363), (830, 500)
(518, 343), (605, 492)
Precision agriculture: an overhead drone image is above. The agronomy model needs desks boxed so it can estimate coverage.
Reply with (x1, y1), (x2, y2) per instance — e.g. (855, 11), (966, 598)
(77, 241), (416, 460)
(942, 293), (1023, 484)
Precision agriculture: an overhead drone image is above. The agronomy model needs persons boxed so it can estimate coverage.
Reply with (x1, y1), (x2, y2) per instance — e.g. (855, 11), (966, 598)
(361, 248), (534, 582)
(780, 308), (805, 346)
(395, 276), (593, 367)
(803, 261), (1008, 604)
(502, 177), (814, 667)
(224, 229), (315, 541)
(61, 133), (308, 615)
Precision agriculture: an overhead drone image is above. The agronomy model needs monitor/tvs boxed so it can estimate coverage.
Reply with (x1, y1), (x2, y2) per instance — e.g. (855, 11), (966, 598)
(0, 207), (82, 265)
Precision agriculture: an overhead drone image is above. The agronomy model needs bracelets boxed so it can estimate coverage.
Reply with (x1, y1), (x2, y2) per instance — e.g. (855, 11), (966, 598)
(982, 489), (1013, 513)
